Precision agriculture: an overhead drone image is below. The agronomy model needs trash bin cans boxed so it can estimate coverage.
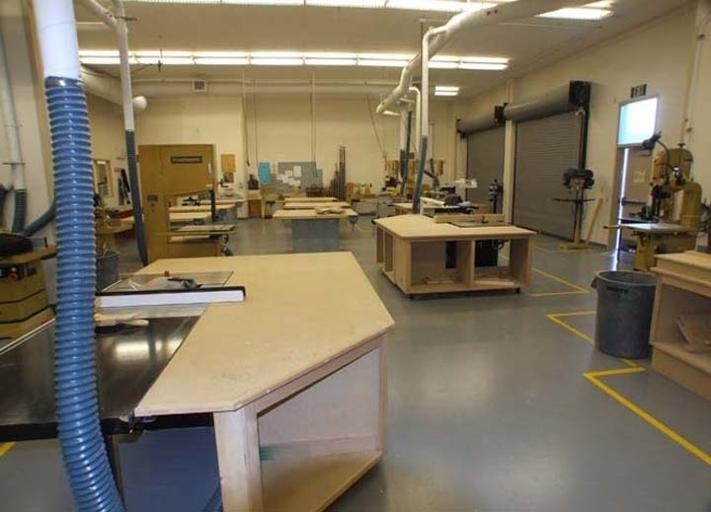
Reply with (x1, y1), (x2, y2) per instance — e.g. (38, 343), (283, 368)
(591, 270), (658, 359)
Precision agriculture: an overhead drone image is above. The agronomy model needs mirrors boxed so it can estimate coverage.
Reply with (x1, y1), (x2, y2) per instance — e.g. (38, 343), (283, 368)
(92, 159), (114, 199)
